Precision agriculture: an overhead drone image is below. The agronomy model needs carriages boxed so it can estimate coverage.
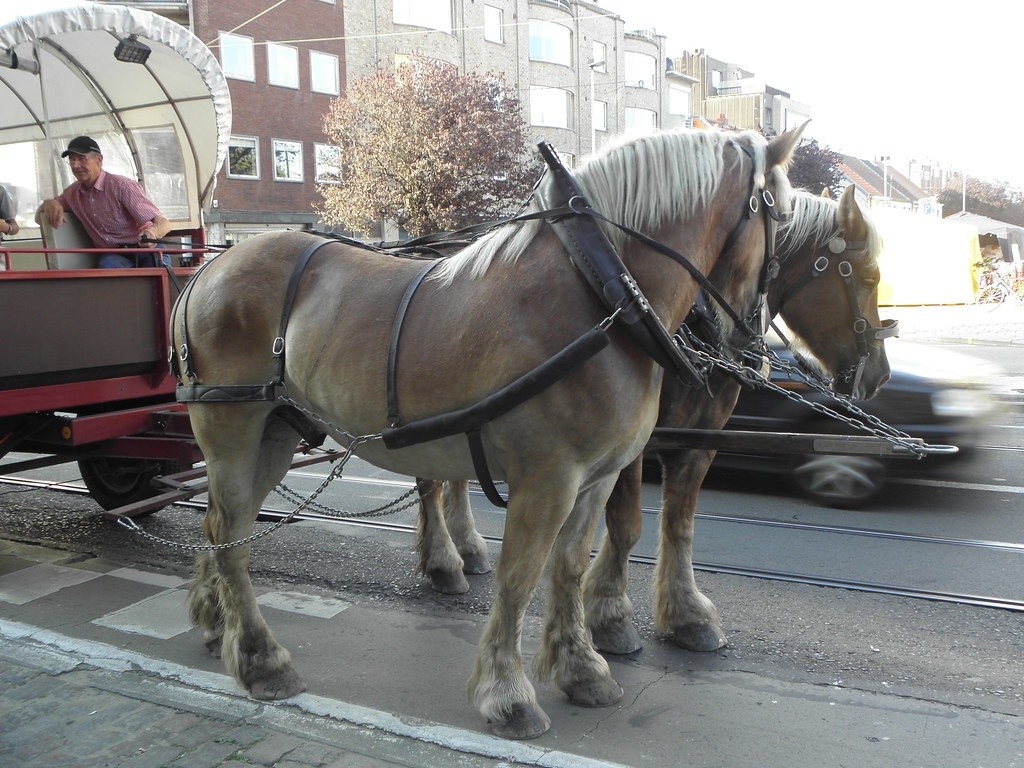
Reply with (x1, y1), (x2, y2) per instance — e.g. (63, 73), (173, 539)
(0, 0), (901, 743)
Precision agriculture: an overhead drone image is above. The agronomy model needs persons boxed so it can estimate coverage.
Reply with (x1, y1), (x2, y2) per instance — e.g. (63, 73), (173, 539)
(33, 136), (173, 270)
(0, 184), (20, 241)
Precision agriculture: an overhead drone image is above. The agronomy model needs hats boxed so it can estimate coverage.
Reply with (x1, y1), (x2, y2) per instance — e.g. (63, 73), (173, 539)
(61, 136), (101, 158)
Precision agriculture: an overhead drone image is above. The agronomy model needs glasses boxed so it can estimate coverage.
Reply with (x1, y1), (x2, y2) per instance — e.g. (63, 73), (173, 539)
(69, 156), (95, 161)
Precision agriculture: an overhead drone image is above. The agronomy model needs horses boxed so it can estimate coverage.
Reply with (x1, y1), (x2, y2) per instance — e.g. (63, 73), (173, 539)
(167, 117), (893, 740)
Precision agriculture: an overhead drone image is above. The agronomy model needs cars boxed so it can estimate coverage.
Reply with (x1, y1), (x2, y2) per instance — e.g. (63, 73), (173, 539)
(642, 338), (1000, 509)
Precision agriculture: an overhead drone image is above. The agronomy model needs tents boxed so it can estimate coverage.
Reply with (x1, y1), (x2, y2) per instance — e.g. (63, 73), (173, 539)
(948, 212), (1024, 262)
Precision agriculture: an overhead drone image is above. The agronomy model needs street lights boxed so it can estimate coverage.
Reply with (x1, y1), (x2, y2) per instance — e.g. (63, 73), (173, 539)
(876, 152), (972, 217)
